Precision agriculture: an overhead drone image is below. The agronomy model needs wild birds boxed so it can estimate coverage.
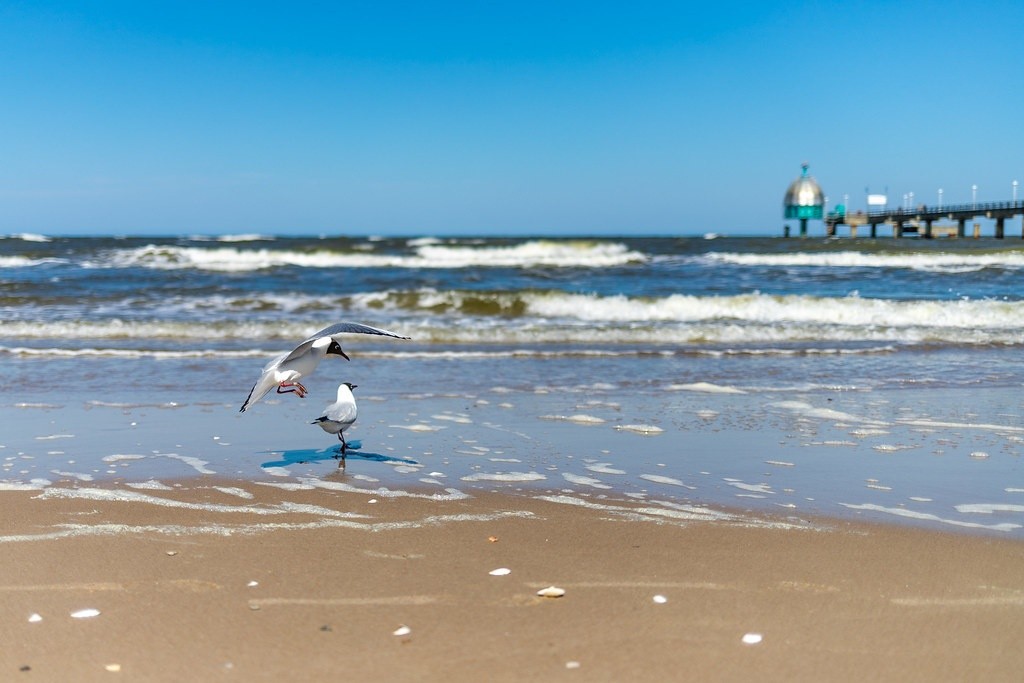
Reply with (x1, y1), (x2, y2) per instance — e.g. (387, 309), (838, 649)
(310, 383), (359, 458)
(239, 323), (411, 412)
